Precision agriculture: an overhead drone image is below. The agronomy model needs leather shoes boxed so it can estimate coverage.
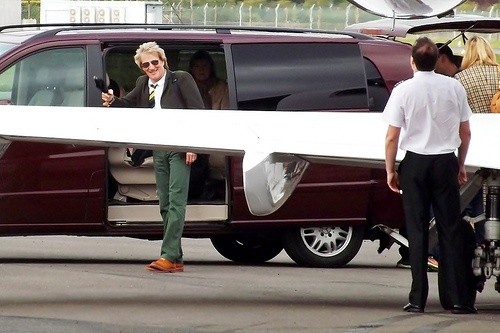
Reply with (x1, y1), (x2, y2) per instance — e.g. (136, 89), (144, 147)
(453, 304), (477, 314)
(403, 303), (424, 312)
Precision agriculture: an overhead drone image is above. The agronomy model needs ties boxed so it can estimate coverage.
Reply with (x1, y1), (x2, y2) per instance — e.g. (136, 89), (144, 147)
(148, 84), (159, 108)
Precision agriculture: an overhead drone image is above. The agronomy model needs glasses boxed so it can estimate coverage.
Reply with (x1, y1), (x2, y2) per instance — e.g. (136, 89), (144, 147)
(140, 60), (161, 68)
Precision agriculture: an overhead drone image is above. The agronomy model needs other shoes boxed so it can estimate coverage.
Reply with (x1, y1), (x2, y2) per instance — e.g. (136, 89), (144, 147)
(149, 259), (184, 272)
(397, 259), (411, 268)
(201, 181), (216, 199)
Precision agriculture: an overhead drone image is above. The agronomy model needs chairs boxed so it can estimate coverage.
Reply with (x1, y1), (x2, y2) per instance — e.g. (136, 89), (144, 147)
(28, 66), (84, 107)
(106, 72), (157, 184)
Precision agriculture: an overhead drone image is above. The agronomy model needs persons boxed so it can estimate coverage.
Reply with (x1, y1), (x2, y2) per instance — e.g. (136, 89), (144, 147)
(383, 36), (500, 314)
(97, 41), (222, 275)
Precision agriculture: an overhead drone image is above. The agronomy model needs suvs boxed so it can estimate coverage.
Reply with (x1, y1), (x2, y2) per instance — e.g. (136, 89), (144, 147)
(0, 18), (500, 264)
